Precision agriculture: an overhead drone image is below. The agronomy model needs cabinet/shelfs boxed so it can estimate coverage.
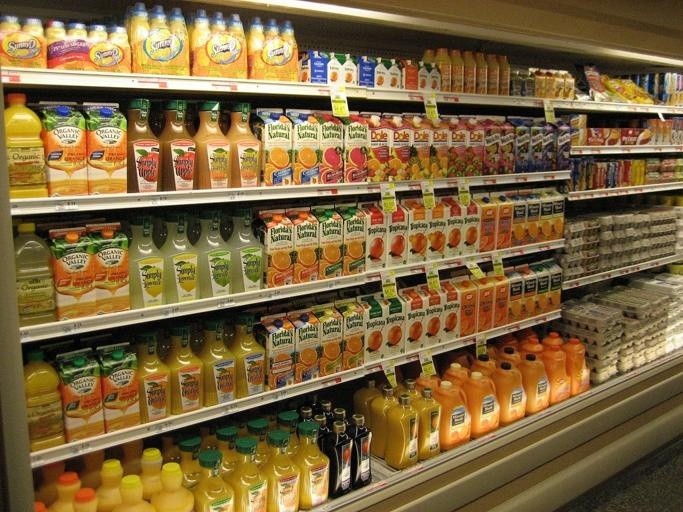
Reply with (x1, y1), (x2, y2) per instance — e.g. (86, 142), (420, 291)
(0, 0), (683, 512)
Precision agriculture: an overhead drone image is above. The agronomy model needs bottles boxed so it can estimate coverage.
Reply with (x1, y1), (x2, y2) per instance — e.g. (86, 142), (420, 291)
(32, 376), (444, 512)
(14, 216), (59, 324)
(125, 211), (261, 307)
(0, 10), (301, 77)
(20, 341), (63, 451)
(0, 95), (45, 200)
(422, 43), (510, 95)
(418, 330), (590, 444)
(134, 313), (267, 421)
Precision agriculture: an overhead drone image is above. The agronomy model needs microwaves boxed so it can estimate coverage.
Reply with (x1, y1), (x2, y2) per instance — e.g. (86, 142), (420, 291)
(128, 93), (263, 192)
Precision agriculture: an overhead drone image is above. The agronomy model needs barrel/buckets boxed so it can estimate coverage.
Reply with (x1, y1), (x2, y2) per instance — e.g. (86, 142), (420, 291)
(497, 345), (523, 366)
(541, 345), (572, 406)
(565, 337), (591, 396)
(443, 362), (470, 387)
(543, 331), (564, 349)
(462, 372), (500, 438)
(491, 362), (526, 427)
(471, 354), (498, 374)
(433, 379), (471, 451)
(518, 337), (543, 356)
(415, 372), (439, 393)
(517, 354), (550, 415)
